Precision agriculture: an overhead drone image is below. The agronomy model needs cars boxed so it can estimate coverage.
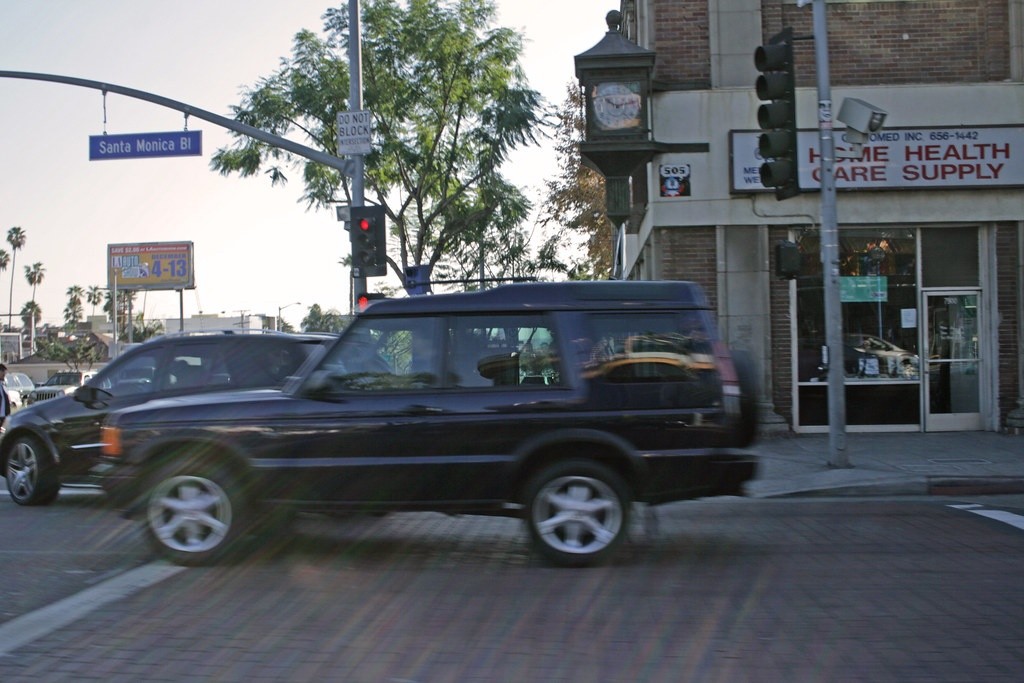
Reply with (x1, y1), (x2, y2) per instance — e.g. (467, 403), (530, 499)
(842, 332), (919, 378)
(116, 366), (156, 395)
(3, 386), (23, 411)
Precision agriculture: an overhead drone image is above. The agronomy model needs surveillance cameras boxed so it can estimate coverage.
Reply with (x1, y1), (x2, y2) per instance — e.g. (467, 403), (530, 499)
(835, 97), (888, 133)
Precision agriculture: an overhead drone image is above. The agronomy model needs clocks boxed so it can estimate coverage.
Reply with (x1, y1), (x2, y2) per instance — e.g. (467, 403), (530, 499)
(592, 80), (642, 133)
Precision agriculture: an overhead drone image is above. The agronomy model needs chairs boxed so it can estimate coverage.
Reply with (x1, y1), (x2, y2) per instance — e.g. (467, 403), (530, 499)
(170, 359), (203, 390)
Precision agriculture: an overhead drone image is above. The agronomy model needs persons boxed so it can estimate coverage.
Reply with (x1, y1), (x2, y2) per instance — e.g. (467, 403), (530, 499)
(0, 364), (10, 429)
(367, 333), (440, 390)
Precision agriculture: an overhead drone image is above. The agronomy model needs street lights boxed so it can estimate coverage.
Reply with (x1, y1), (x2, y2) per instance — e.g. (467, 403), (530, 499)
(279, 303), (302, 332)
(114, 262), (148, 362)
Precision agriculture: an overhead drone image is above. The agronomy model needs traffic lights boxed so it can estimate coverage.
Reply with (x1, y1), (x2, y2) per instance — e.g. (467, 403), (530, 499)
(350, 205), (387, 277)
(754, 27), (798, 201)
(357, 292), (384, 312)
(774, 244), (801, 277)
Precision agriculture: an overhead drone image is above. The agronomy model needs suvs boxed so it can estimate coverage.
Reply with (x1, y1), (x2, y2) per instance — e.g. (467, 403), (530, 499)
(98, 280), (764, 568)
(1, 383), (36, 401)
(0, 327), (393, 506)
(26, 369), (113, 406)
